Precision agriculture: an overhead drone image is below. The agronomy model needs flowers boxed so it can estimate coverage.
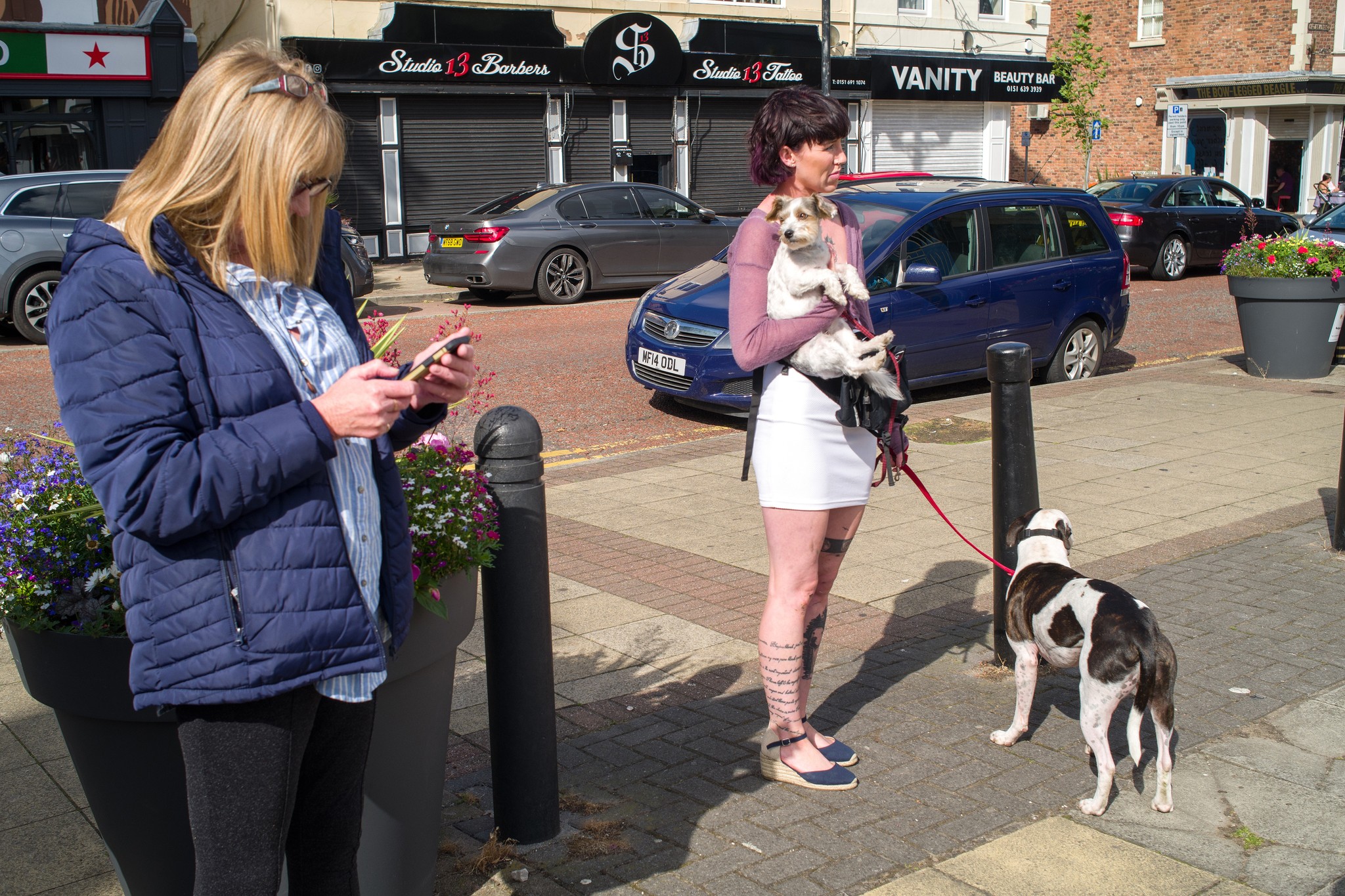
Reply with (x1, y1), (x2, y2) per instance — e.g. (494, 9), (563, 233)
(0, 306), (503, 638)
(1218, 205), (1345, 282)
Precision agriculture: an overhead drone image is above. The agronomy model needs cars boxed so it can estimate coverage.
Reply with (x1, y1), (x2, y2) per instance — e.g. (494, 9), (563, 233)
(1286, 200), (1345, 252)
(422, 181), (747, 305)
(1066, 174), (1301, 282)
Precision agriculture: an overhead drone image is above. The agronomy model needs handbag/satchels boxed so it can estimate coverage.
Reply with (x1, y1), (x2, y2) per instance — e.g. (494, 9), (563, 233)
(1314, 195), (1320, 208)
(797, 330), (911, 429)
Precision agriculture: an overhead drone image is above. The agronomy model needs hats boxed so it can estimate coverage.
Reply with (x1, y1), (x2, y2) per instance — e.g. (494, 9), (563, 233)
(1172, 164), (1181, 174)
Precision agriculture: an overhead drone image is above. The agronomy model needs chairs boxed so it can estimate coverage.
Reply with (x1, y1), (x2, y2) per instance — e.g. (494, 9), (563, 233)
(923, 247), (968, 276)
(1277, 181), (1299, 213)
(993, 240), (1045, 269)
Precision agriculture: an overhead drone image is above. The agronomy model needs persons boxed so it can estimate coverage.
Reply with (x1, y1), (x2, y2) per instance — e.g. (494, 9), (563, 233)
(1318, 173), (1340, 194)
(48, 49), (474, 896)
(724, 85), (907, 788)
(1270, 168), (1294, 212)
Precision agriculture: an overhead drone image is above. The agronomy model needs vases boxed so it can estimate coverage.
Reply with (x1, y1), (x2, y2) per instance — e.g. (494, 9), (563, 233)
(1227, 274), (1345, 380)
(1, 559), (479, 896)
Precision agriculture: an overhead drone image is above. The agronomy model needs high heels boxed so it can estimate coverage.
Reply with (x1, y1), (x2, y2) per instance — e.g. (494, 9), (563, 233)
(759, 721), (859, 790)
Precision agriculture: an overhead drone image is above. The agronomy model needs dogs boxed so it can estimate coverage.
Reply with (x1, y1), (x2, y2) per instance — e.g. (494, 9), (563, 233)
(762, 193), (904, 402)
(991, 506), (1178, 814)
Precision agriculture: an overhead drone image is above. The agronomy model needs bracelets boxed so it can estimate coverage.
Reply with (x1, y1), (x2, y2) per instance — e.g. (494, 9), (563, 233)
(1276, 189), (1279, 192)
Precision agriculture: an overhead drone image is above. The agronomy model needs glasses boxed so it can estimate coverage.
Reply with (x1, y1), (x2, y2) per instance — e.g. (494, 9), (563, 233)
(1330, 177), (1332, 178)
(1172, 172), (1177, 174)
(299, 177), (332, 196)
(250, 73), (329, 105)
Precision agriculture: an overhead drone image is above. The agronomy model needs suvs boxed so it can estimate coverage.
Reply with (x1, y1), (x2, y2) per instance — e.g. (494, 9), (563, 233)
(625, 176), (1132, 418)
(0, 168), (374, 345)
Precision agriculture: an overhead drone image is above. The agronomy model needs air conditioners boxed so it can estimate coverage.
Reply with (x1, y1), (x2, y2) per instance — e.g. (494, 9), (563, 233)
(1026, 103), (1052, 120)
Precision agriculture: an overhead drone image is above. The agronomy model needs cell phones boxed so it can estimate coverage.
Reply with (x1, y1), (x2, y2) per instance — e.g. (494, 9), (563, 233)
(401, 336), (471, 382)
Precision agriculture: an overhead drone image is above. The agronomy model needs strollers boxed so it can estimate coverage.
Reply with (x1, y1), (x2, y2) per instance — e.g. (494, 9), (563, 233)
(1314, 183), (1345, 215)
